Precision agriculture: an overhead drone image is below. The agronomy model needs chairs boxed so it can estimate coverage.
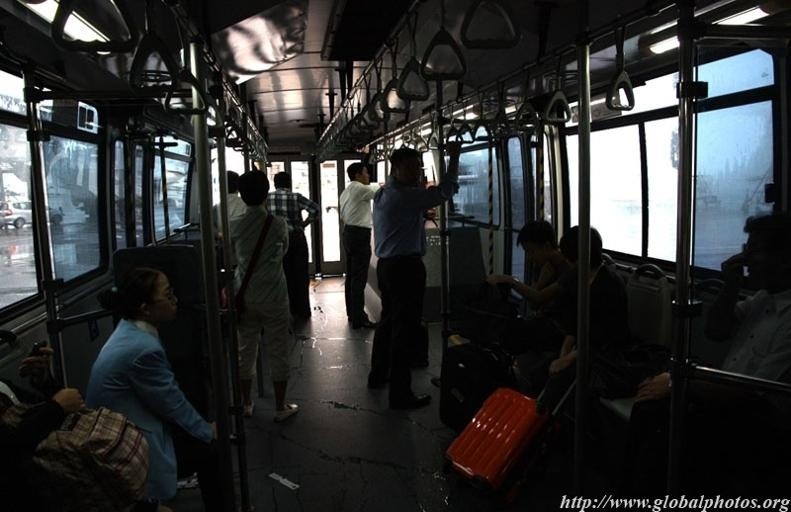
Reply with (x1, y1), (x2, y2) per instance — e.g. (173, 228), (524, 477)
(548, 239), (788, 503)
(0, 203), (266, 512)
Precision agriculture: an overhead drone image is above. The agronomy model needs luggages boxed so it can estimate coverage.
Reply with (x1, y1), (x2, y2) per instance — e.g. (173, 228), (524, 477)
(443, 345), (578, 483)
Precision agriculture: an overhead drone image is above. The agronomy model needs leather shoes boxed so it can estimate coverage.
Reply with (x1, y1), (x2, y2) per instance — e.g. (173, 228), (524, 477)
(274, 404), (298, 421)
(351, 317), (378, 329)
(407, 360), (441, 408)
(243, 399), (253, 417)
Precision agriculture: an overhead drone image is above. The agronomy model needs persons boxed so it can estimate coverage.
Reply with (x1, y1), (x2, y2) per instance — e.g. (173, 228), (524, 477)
(0, 140), (791, 512)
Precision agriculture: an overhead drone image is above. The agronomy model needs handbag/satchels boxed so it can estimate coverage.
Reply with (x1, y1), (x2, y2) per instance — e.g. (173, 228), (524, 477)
(232, 288), (248, 321)
(32, 397), (150, 511)
